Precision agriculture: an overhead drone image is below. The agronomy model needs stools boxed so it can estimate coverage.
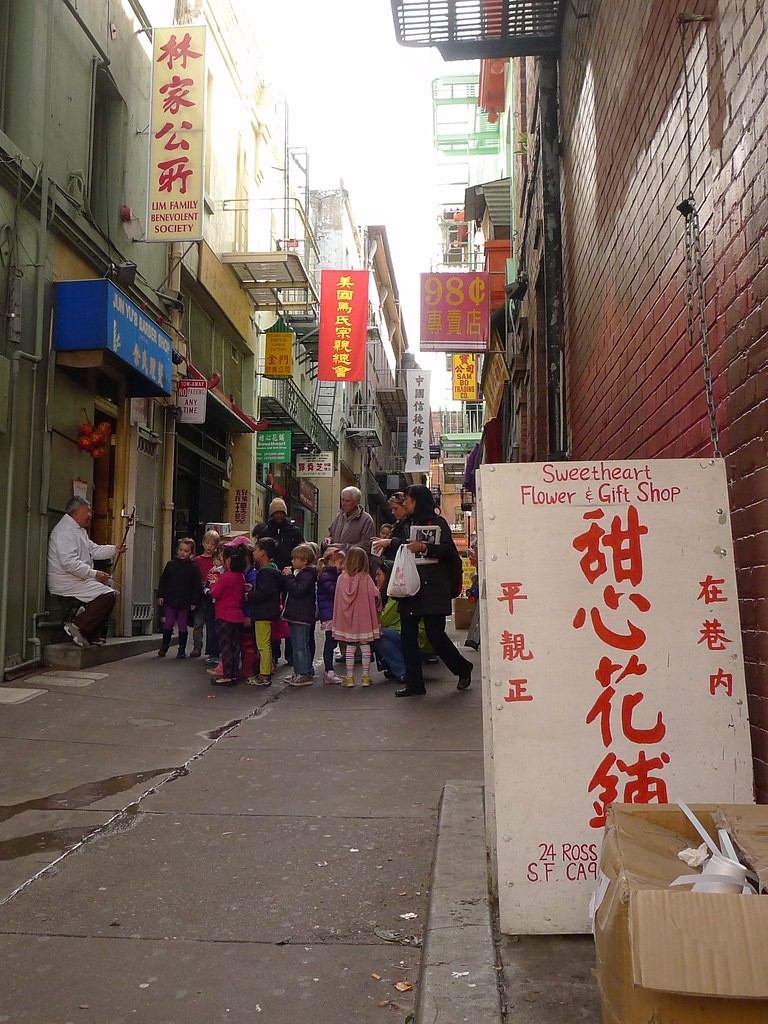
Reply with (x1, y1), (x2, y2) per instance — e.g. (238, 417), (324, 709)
(46, 594), (85, 644)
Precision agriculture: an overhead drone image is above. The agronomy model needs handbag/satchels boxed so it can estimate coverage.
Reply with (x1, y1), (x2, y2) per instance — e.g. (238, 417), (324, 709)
(386, 544), (420, 597)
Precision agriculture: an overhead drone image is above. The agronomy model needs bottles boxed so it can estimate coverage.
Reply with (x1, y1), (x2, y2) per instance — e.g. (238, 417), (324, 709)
(206, 564), (216, 588)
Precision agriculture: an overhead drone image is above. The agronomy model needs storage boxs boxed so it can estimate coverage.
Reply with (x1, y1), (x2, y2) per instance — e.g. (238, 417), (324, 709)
(451, 596), (476, 629)
(591, 802), (768, 1024)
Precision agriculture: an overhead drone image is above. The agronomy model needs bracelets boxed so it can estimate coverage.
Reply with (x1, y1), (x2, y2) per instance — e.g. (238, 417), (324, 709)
(421, 542), (426, 553)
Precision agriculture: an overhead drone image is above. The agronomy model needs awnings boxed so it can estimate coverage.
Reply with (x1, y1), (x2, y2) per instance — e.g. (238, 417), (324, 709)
(50, 277), (172, 398)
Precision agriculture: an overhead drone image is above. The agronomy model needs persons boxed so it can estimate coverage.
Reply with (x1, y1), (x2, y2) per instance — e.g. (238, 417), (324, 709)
(261, 495), (306, 665)
(278, 542), (319, 686)
(370, 483), (474, 697)
(204, 540), (230, 675)
(47, 495), (128, 646)
(332, 547), (382, 687)
(190, 530), (218, 658)
(322, 487), (375, 663)
(252, 523), (265, 538)
(207, 546), (245, 686)
(225, 536), (290, 687)
(420, 529), (434, 558)
(176, 511), (187, 531)
(156, 538), (202, 658)
(316, 545), (345, 684)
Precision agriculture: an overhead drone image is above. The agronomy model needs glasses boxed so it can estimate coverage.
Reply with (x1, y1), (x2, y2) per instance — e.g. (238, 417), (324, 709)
(381, 561), (389, 568)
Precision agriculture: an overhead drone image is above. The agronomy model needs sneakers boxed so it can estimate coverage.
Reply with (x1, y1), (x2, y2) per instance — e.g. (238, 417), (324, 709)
(341, 678), (355, 688)
(283, 674), (296, 683)
(207, 650), (438, 675)
(290, 676), (313, 686)
(246, 676), (271, 685)
(323, 670), (342, 685)
(88, 631), (104, 645)
(64, 623), (90, 648)
(361, 675), (372, 687)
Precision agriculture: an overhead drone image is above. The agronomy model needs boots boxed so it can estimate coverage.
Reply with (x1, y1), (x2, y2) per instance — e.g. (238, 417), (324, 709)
(158, 629), (173, 656)
(177, 631), (189, 657)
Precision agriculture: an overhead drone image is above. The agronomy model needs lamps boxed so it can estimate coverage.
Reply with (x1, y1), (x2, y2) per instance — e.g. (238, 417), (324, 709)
(111, 259), (137, 286)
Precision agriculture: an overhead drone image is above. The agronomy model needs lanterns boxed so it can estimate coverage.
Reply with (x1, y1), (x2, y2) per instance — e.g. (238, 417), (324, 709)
(77, 422), (112, 459)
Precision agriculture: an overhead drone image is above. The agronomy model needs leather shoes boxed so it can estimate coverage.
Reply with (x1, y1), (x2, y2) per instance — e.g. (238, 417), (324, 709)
(457, 663), (473, 689)
(395, 688), (427, 698)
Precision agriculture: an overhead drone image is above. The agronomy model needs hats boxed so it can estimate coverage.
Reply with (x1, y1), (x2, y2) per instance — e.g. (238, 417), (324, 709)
(269, 498), (288, 516)
(223, 536), (251, 548)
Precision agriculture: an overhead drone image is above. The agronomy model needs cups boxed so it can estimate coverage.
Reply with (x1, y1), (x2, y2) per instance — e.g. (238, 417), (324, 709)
(371, 541), (384, 556)
(691, 854), (747, 892)
(285, 567), (291, 570)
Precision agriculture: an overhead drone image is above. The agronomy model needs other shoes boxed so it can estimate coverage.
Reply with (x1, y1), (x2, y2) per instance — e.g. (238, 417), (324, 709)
(229, 678), (238, 685)
(191, 648), (201, 657)
(210, 679), (232, 686)
